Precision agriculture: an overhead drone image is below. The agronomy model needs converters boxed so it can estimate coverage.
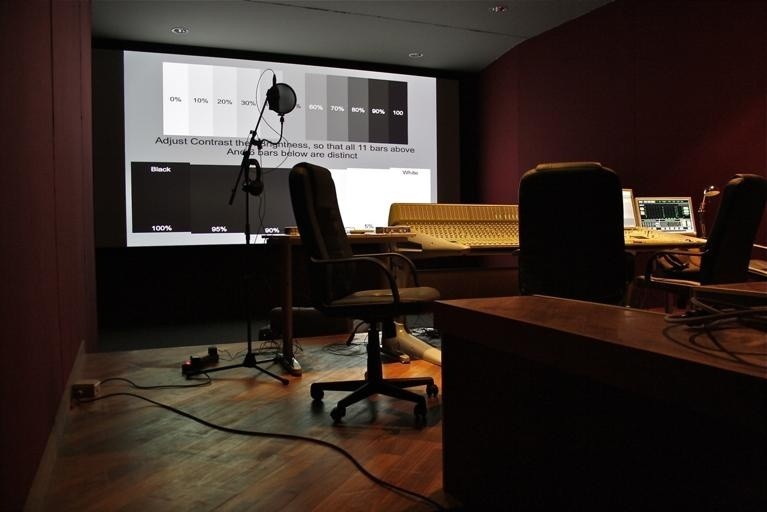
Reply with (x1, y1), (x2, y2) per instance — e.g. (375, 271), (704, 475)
(74, 378), (102, 397)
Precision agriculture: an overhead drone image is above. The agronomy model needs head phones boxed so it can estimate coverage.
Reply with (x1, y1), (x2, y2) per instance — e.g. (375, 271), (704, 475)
(242, 158), (265, 196)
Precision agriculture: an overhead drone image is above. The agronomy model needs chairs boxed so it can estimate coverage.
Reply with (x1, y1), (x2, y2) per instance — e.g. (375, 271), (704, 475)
(510, 163), (634, 304)
(289, 163), (439, 427)
(634, 173), (767, 307)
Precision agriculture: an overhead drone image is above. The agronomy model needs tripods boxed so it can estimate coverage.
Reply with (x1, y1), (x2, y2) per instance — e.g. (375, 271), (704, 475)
(189, 159), (291, 387)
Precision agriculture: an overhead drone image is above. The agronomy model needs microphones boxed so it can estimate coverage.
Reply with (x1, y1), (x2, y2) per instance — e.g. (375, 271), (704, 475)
(268, 75), (280, 111)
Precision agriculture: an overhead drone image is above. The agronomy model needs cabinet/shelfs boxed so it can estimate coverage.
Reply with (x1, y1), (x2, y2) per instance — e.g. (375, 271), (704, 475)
(434, 292), (767, 511)
(695, 281), (767, 327)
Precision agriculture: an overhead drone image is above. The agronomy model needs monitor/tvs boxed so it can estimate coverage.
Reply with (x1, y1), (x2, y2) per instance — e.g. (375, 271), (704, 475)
(634, 196), (697, 236)
(622, 189), (636, 230)
